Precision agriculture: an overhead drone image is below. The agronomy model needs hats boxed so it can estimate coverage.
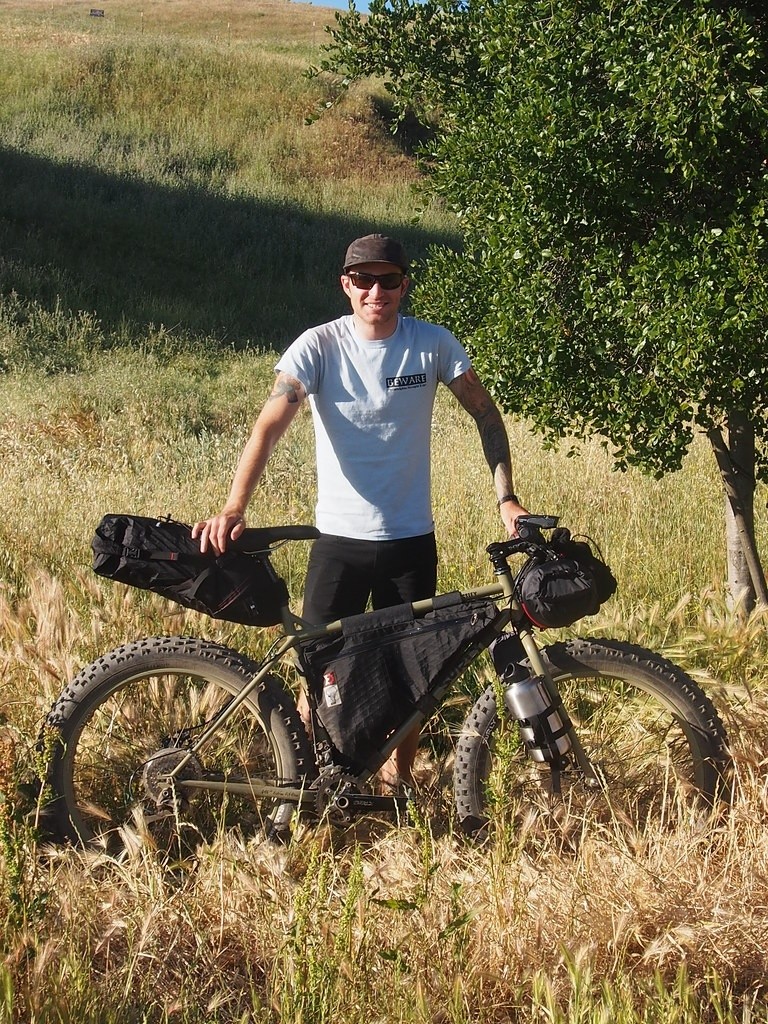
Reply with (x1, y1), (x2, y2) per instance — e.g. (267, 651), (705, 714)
(342, 235), (409, 272)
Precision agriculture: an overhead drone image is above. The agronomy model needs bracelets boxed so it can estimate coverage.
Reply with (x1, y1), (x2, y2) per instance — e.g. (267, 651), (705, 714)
(497, 495), (519, 514)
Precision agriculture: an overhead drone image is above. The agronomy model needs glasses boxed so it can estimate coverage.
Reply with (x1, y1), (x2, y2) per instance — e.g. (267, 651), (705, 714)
(347, 272), (407, 290)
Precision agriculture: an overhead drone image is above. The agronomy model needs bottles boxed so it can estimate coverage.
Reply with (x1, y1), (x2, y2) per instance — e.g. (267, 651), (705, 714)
(504, 661), (572, 764)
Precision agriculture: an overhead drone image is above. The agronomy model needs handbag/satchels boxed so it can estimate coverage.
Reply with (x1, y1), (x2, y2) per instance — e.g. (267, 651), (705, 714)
(519, 527), (617, 630)
(90, 514), (289, 628)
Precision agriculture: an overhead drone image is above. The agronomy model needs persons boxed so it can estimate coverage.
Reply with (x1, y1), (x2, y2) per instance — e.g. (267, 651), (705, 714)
(190, 234), (532, 823)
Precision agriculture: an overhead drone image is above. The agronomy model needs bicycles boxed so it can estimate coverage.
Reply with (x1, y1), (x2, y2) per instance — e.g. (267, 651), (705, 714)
(33, 514), (736, 895)
